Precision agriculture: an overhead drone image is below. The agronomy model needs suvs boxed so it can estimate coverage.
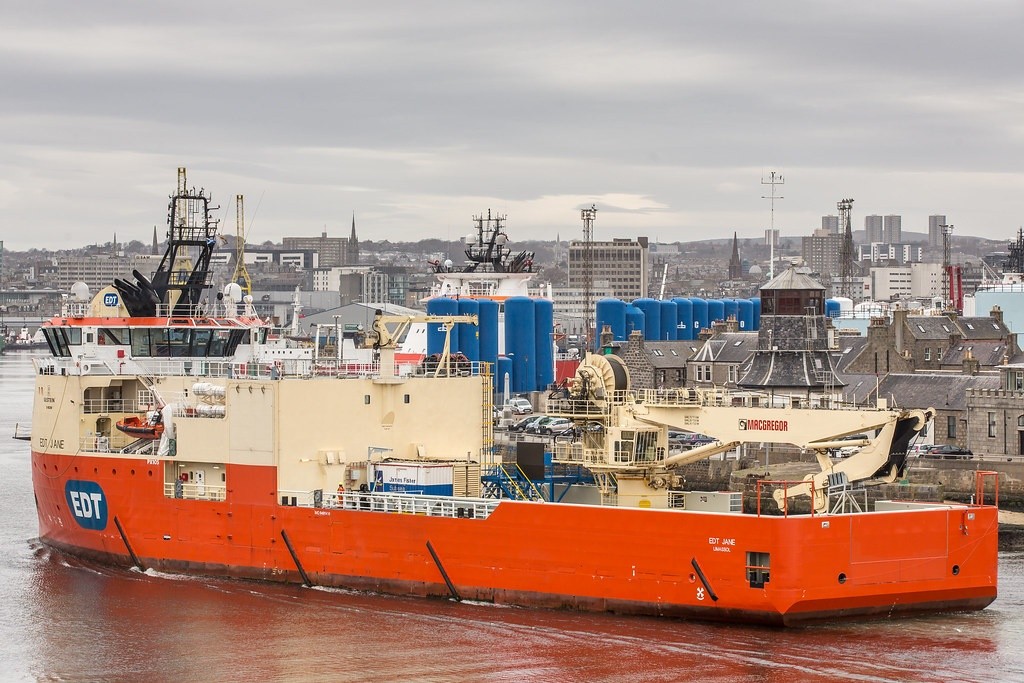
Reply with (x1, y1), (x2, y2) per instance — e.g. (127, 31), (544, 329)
(510, 396), (534, 415)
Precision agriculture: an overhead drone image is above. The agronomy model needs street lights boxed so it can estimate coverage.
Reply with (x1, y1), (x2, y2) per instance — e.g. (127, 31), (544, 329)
(508, 352), (528, 398)
(332, 314), (342, 368)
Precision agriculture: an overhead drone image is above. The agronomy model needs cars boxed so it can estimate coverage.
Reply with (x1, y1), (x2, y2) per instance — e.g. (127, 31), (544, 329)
(924, 444), (974, 459)
(907, 444), (935, 458)
(667, 431), (718, 450)
(493, 405), (605, 437)
(829, 434), (871, 457)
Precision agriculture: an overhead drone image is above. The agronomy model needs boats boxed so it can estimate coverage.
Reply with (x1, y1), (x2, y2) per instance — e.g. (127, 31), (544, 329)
(10, 166), (1002, 628)
(116, 416), (166, 440)
